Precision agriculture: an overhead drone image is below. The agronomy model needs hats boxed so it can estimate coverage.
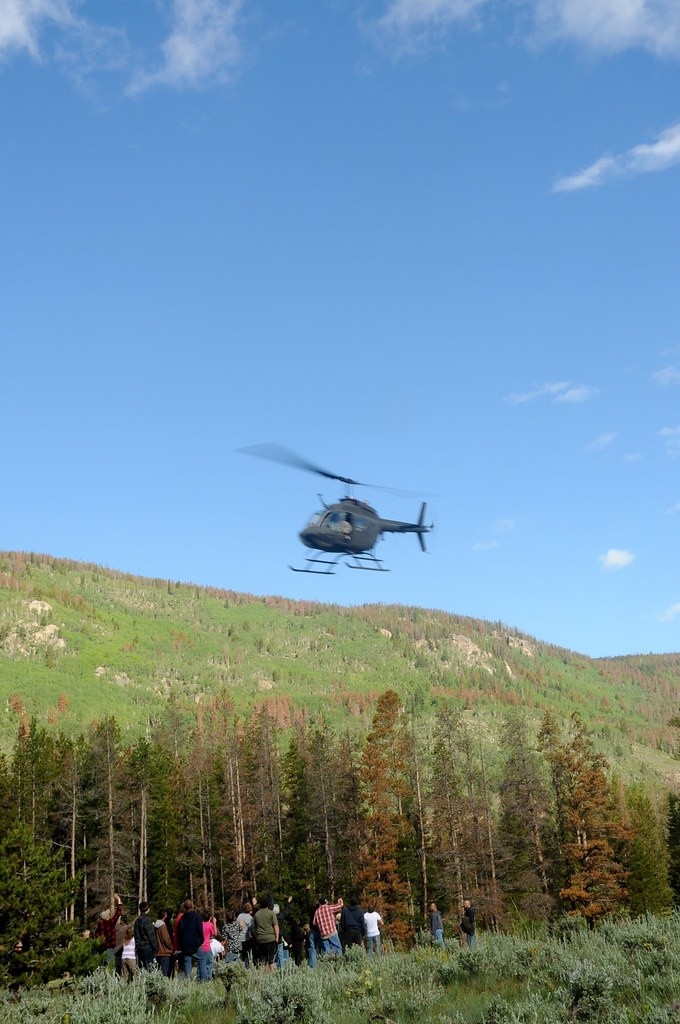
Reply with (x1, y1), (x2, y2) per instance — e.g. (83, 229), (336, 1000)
(139, 902), (150, 913)
(100, 908), (110, 919)
(272, 904), (279, 915)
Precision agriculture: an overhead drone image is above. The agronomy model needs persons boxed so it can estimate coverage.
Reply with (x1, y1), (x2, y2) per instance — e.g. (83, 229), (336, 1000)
(461, 899), (476, 951)
(252, 895), (293, 969)
(291, 915), (306, 967)
(429, 902), (446, 950)
(253, 896), (279, 976)
(334, 512), (352, 543)
(235, 902), (256, 969)
(221, 909), (244, 965)
(340, 896), (365, 951)
(363, 903), (384, 959)
(302, 924), (319, 969)
(282, 927), (292, 962)
(312, 896), (344, 957)
(8, 891), (226, 992)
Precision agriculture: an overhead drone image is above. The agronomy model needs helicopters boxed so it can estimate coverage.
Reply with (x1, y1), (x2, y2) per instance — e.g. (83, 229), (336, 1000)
(241, 443), (435, 574)
